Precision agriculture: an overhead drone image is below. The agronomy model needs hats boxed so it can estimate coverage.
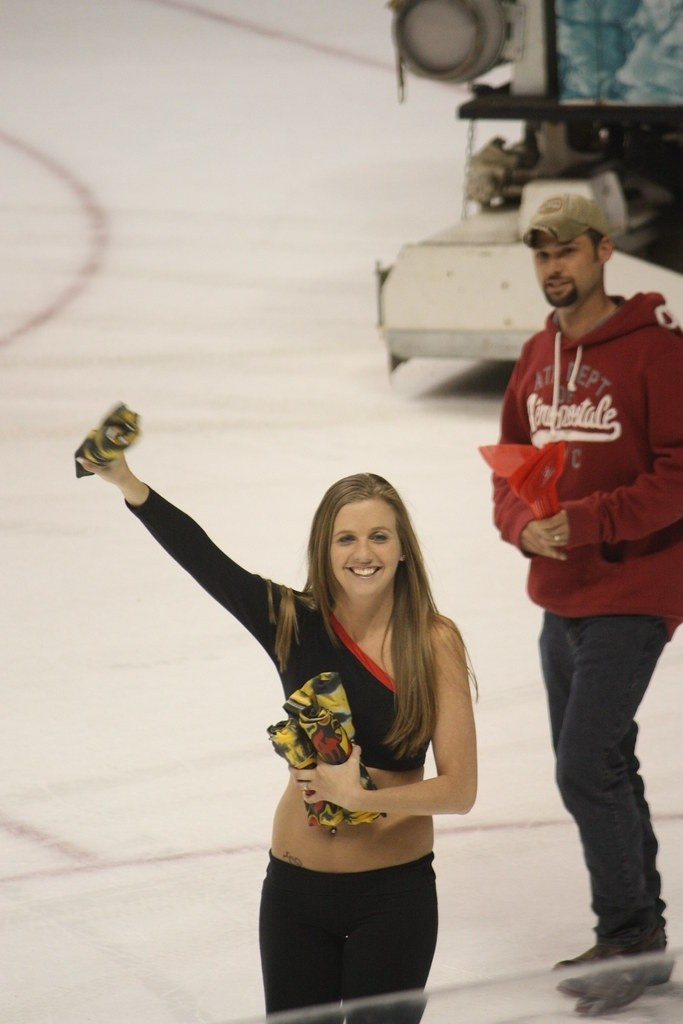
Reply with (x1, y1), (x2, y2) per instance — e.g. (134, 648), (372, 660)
(523, 193), (609, 248)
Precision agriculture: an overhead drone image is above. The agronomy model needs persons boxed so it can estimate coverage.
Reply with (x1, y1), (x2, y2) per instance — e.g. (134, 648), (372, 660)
(76, 449), (477, 1024)
(493, 194), (683, 1014)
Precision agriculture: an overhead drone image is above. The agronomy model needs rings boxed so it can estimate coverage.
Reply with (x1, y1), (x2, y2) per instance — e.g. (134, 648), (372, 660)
(555, 534), (559, 541)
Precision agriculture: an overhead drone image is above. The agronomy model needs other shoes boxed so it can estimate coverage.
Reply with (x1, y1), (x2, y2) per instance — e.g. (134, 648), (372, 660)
(554, 945), (674, 1013)
